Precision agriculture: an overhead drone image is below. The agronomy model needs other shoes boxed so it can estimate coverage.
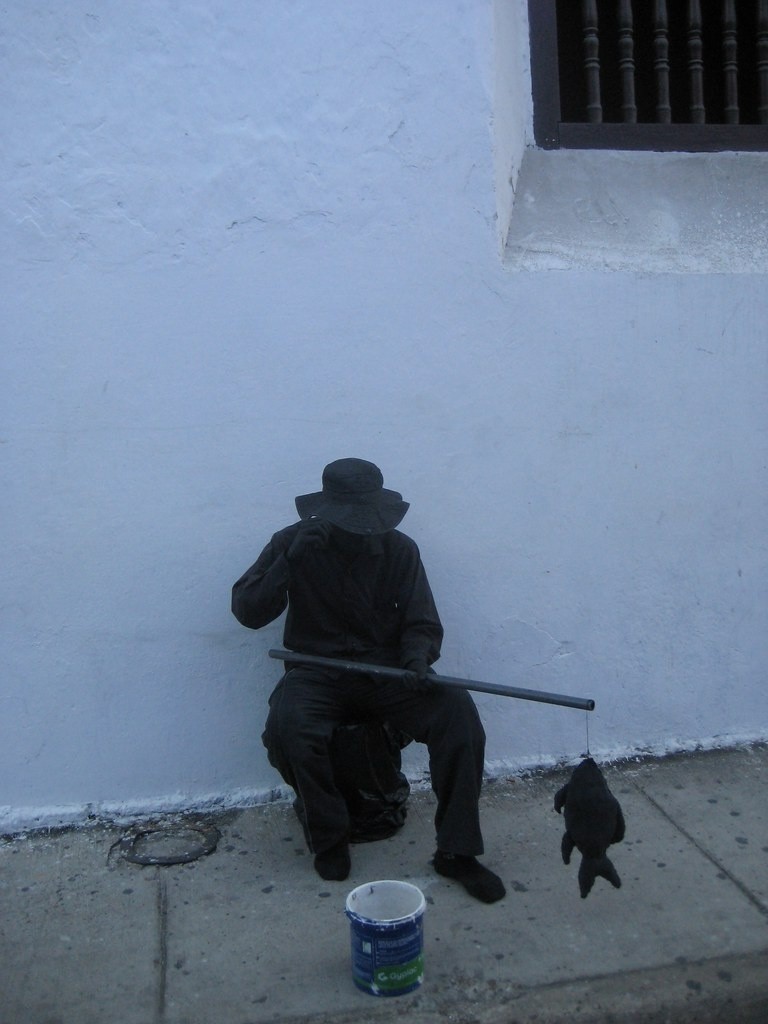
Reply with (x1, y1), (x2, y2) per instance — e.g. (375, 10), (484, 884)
(314, 839), (351, 881)
(434, 849), (506, 903)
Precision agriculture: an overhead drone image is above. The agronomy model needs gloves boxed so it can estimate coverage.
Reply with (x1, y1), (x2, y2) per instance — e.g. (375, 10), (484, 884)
(403, 660), (436, 691)
(286, 517), (332, 567)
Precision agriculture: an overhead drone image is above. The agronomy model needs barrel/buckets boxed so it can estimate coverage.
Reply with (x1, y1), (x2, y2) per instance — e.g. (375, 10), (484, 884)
(343, 879), (427, 998)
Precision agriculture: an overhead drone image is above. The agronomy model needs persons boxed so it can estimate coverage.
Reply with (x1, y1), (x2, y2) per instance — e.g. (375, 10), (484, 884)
(231, 457), (507, 905)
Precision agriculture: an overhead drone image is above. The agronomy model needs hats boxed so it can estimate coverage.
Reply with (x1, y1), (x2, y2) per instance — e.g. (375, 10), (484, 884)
(295, 458), (410, 536)
